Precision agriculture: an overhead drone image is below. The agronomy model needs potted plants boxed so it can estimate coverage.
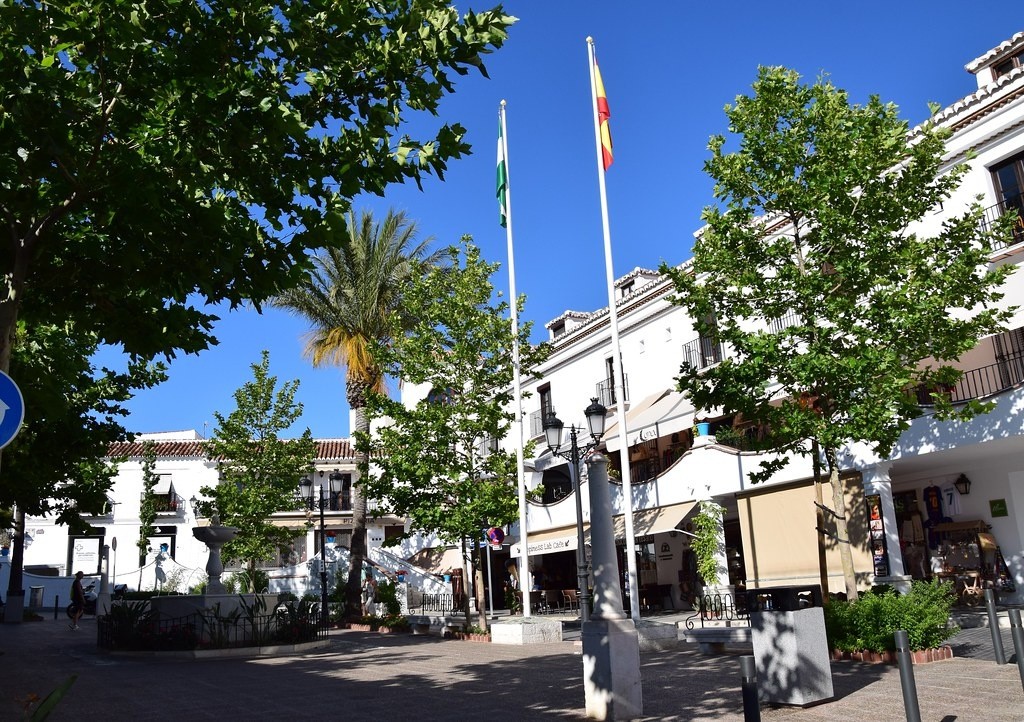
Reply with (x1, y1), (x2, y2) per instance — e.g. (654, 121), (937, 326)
(443, 573), (451, 582)
(459, 626), (490, 642)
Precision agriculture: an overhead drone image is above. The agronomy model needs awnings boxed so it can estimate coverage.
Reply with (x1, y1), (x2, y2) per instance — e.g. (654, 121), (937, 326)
(509, 499), (704, 559)
(530, 257), (1024, 472)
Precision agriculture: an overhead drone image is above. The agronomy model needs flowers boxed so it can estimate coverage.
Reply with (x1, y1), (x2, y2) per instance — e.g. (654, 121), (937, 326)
(1, 546), (9, 549)
(325, 531), (337, 537)
(395, 570), (408, 575)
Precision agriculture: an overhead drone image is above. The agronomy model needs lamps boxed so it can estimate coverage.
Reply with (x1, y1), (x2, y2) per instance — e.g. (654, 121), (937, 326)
(953, 473), (971, 495)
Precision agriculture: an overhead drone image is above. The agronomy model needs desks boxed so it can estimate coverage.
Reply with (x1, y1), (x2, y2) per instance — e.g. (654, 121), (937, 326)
(638, 584), (674, 615)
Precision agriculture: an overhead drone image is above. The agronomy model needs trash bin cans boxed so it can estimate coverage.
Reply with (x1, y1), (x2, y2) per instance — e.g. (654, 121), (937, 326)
(4, 589), (25, 624)
(745, 584), (835, 706)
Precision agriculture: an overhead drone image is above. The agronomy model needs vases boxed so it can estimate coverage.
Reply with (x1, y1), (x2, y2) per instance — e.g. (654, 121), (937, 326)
(1, 549), (9, 556)
(327, 537), (335, 543)
(696, 423), (710, 436)
(398, 575), (404, 582)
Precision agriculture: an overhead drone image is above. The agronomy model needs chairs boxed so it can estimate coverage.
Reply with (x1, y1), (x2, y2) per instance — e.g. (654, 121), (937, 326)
(514, 590), (581, 616)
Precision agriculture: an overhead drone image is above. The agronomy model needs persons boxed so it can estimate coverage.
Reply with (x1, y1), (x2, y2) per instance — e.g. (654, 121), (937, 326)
(68, 571), (93, 631)
(361, 572), (378, 616)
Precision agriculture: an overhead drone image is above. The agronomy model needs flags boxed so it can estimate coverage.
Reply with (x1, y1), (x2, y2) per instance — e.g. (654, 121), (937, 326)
(590, 42), (614, 172)
(496, 105), (507, 228)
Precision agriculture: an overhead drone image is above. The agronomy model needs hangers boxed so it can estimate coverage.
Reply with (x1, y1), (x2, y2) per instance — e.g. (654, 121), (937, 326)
(928, 478), (951, 487)
